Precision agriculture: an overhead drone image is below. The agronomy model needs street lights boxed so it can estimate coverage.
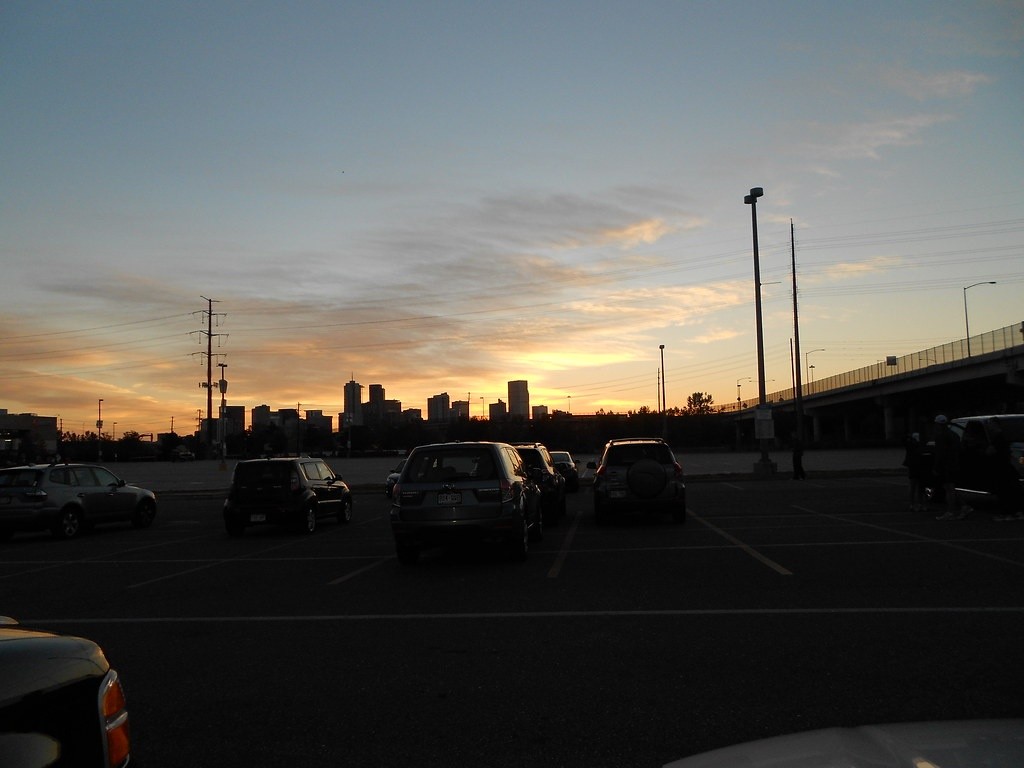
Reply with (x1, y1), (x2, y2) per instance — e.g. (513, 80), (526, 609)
(962, 279), (998, 358)
(743, 187), (775, 452)
(736, 377), (752, 411)
(805, 347), (826, 395)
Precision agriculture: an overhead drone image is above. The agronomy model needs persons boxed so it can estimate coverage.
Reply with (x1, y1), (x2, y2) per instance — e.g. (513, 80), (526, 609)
(789, 432), (807, 481)
(905, 414), (1021, 523)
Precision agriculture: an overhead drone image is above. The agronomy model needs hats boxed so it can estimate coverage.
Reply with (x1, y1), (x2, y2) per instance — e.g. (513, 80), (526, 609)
(935, 415), (947, 425)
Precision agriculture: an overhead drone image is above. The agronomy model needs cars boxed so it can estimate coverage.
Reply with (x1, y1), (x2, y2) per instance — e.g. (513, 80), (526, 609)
(0, 461), (159, 540)
(385, 447), (581, 504)
(587, 435), (689, 524)
(0, 615), (132, 767)
(388, 440), (546, 560)
(926, 414), (1024, 515)
(223, 455), (353, 538)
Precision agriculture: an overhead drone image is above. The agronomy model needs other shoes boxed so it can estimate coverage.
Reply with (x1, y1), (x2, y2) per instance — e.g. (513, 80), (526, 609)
(936, 512), (955, 521)
(956, 506), (974, 518)
(911, 503), (927, 512)
